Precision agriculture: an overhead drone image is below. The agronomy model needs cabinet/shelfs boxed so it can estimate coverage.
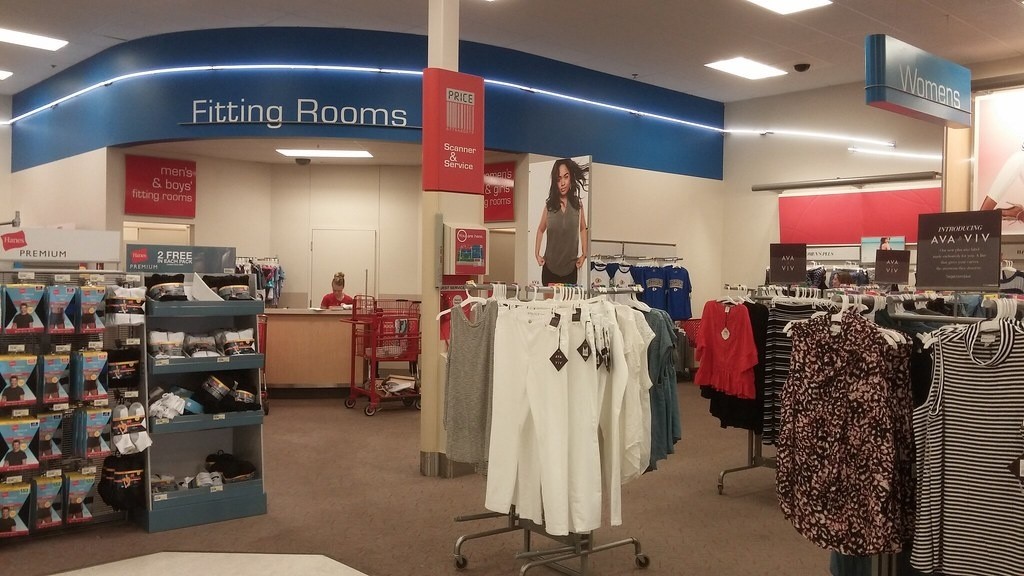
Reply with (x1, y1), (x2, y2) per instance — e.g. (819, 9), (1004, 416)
(0, 260), (146, 542)
(145, 294), (268, 534)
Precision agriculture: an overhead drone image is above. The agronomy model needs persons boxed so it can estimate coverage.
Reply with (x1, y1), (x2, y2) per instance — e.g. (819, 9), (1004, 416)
(0, 303), (33, 533)
(534, 159), (589, 288)
(879, 238), (892, 250)
(320, 272), (353, 309)
(980, 152), (1024, 224)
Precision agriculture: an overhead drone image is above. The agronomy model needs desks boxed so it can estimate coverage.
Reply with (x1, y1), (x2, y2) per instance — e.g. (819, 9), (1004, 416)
(264, 308), (369, 388)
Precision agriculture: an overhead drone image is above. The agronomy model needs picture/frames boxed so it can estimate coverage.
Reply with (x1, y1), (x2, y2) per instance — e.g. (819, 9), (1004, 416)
(861, 236), (905, 263)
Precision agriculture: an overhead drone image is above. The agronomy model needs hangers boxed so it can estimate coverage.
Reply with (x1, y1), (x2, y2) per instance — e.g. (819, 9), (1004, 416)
(235, 253), (280, 269)
(436, 252), (688, 321)
(704, 260), (1023, 350)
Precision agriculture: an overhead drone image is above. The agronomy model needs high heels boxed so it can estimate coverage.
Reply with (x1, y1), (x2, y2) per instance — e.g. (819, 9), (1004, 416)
(994, 201), (1024, 227)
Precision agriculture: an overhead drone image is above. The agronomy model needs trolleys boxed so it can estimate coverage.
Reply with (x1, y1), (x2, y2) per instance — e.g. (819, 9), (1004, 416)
(257, 315), (270, 415)
(339, 295), (422, 416)
